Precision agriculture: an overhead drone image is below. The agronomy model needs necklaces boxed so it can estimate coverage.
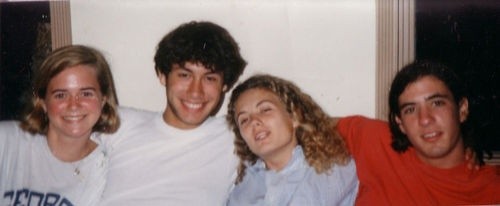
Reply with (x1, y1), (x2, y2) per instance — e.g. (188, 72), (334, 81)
(54, 140), (93, 176)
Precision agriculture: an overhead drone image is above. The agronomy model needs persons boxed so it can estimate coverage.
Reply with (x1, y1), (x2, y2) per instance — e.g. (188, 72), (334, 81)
(225, 74), (362, 206)
(330, 59), (499, 205)
(0, 44), (122, 206)
(87, 19), (249, 206)
